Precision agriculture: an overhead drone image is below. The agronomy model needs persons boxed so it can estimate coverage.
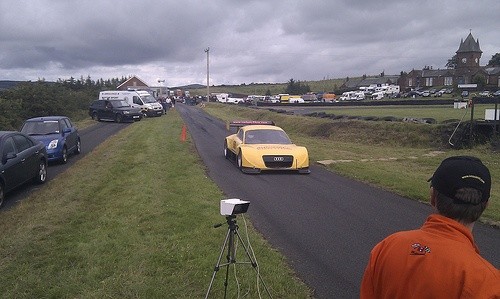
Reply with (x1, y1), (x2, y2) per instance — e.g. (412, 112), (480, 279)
(360, 155), (500, 299)
(155, 94), (216, 111)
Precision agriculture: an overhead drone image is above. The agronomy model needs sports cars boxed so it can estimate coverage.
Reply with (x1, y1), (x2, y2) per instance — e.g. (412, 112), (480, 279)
(224, 121), (311, 174)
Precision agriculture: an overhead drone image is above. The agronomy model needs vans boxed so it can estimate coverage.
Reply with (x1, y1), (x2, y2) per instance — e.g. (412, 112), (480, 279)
(339, 87), (400, 100)
(99, 91), (164, 117)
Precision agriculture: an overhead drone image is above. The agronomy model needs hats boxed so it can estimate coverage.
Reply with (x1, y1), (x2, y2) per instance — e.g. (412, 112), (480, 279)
(428, 156), (491, 205)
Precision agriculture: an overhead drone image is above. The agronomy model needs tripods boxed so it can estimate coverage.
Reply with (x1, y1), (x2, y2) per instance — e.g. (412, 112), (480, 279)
(205, 216), (273, 299)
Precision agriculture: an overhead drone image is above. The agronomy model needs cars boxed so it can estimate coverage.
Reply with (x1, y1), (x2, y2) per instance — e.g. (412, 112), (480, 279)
(392, 88), (500, 98)
(0, 131), (48, 207)
(88, 100), (141, 123)
(19, 116), (81, 163)
